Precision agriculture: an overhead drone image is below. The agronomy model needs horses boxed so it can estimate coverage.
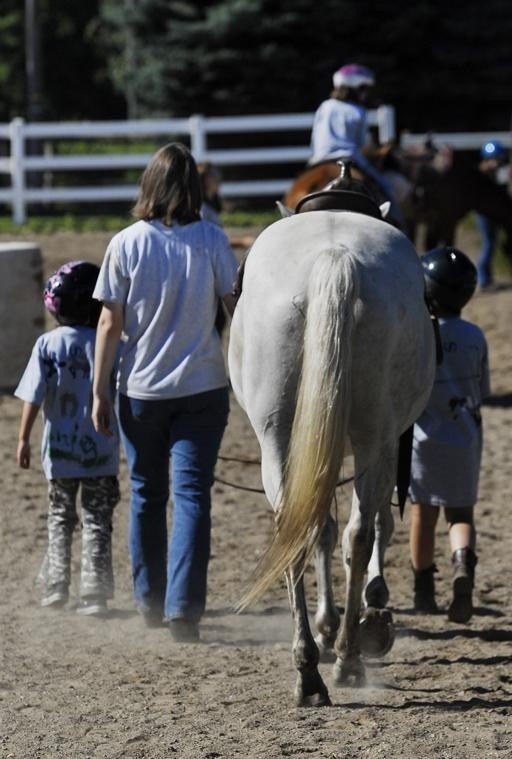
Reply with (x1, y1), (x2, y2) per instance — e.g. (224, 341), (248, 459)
(284, 142), (419, 248)
(225, 198), (440, 710)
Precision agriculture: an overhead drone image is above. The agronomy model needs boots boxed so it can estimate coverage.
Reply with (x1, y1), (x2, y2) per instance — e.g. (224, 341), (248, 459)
(447, 547), (477, 622)
(412, 564), (438, 611)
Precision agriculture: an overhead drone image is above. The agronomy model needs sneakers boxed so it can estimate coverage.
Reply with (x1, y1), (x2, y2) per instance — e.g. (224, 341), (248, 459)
(144, 610), (164, 628)
(40, 584), (67, 608)
(70, 598), (108, 618)
(170, 621), (200, 642)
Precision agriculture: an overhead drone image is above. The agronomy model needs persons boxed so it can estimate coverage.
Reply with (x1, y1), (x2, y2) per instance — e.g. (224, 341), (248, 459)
(472, 139), (504, 289)
(306, 63), (403, 230)
(194, 161), (226, 230)
(91, 143), (240, 644)
(10, 259), (126, 616)
(402, 246), (490, 624)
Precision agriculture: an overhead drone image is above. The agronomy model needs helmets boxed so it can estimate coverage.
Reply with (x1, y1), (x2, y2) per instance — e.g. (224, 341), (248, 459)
(484, 140), (502, 159)
(334, 65), (375, 90)
(44, 261), (100, 324)
(419, 249), (478, 321)
